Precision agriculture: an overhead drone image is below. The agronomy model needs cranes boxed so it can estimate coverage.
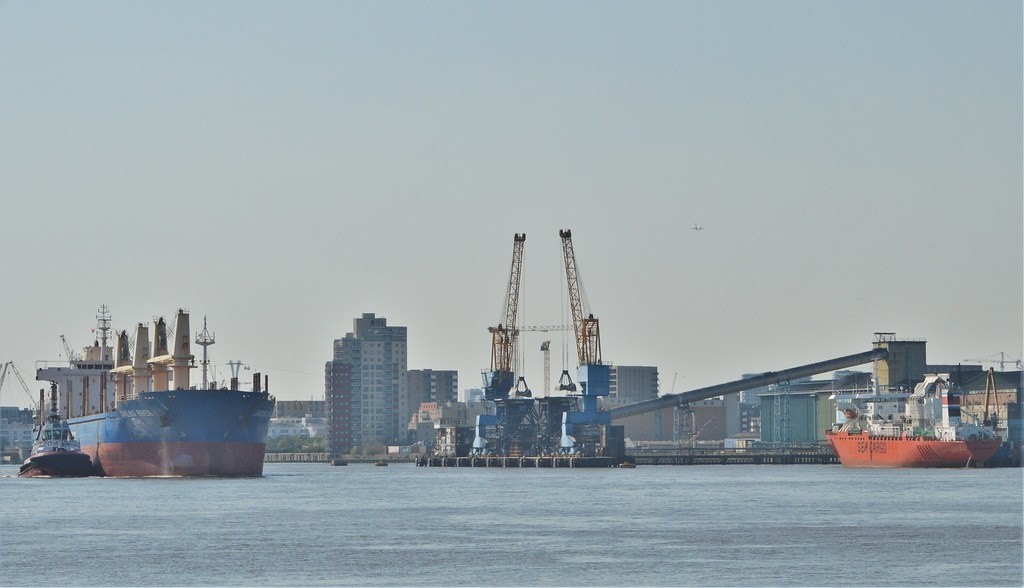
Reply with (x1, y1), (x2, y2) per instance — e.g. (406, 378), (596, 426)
(541, 227), (617, 459)
(473, 232), (535, 456)
(0, 361), (37, 406)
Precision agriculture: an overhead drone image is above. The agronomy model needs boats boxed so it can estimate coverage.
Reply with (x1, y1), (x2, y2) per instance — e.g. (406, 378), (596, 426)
(36, 302), (276, 478)
(823, 367), (1002, 469)
(18, 383), (92, 478)
